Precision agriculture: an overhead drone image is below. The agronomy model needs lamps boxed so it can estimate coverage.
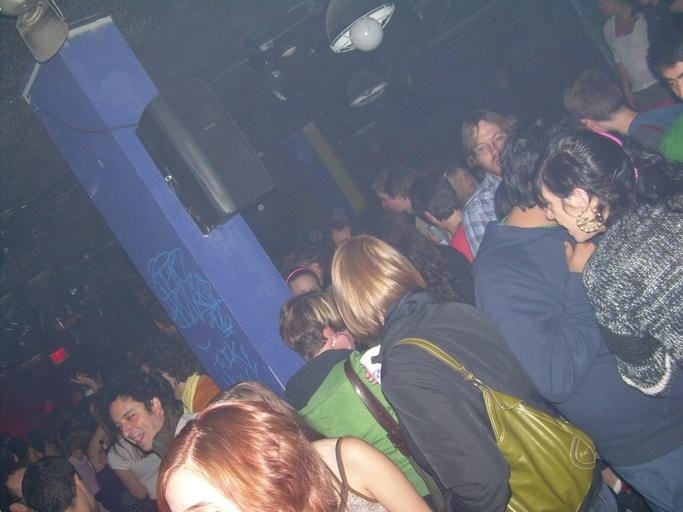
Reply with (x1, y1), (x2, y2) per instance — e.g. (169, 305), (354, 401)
(348, 71), (389, 108)
(326, 0), (395, 52)
(275, 38), (296, 57)
(1, 0), (69, 62)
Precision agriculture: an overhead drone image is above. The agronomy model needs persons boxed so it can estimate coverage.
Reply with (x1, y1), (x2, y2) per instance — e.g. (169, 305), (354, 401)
(2, 4), (683, 512)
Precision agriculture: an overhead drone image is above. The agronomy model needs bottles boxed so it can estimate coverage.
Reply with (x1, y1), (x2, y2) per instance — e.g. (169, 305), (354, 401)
(98, 439), (110, 455)
(427, 224), (450, 247)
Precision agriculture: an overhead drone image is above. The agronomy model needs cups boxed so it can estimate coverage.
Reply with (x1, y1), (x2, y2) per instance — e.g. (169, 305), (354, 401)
(359, 344), (381, 386)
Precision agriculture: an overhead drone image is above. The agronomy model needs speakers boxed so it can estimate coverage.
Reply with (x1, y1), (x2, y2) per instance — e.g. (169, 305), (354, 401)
(134, 80), (274, 233)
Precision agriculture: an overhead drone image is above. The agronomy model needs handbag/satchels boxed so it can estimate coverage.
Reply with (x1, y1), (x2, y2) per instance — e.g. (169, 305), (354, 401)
(482, 386), (597, 512)
